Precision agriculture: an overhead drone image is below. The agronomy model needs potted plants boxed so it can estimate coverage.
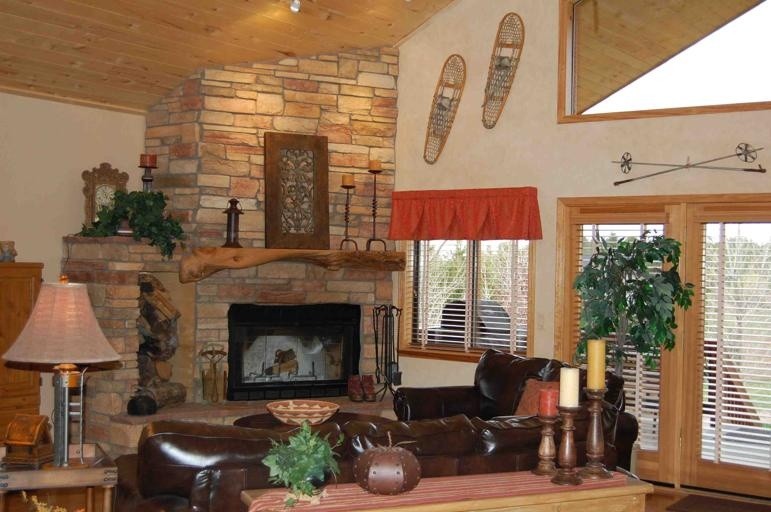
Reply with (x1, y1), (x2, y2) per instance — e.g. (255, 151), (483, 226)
(264, 423), (344, 506)
(74, 188), (189, 269)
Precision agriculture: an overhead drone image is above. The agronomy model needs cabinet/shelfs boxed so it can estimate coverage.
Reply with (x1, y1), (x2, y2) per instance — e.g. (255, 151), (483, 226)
(0, 258), (43, 445)
(0, 444), (118, 512)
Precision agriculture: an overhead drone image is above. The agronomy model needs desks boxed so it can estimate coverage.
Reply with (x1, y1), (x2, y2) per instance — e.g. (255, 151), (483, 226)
(240, 466), (655, 512)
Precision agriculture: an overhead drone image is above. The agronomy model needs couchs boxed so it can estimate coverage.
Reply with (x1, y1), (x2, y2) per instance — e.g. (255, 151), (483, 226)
(114, 410), (639, 512)
(417, 302), (470, 350)
(470, 300), (528, 348)
(393, 348), (626, 421)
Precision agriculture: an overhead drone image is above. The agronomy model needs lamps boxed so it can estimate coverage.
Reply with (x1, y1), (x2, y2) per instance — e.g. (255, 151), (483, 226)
(2, 283), (122, 467)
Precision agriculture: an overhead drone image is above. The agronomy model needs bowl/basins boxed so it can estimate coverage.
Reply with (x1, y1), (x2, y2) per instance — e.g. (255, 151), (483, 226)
(265, 399), (339, 426)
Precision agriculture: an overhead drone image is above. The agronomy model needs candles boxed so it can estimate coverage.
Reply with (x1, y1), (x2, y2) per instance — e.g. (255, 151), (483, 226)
(342, 174), (356, 185)
(140, 154), (160, 166)
(585, 336), (605, 392)
(539, 386), (556, 422)
(558, 365), (579, 409)
(367, 159), (385, 170)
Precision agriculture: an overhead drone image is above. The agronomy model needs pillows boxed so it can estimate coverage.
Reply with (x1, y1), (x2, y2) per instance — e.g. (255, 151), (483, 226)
(513, 378), (560, 417)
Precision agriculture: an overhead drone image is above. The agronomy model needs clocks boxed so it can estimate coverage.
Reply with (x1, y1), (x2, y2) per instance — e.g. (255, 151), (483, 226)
(81, 161), (128, 231)
